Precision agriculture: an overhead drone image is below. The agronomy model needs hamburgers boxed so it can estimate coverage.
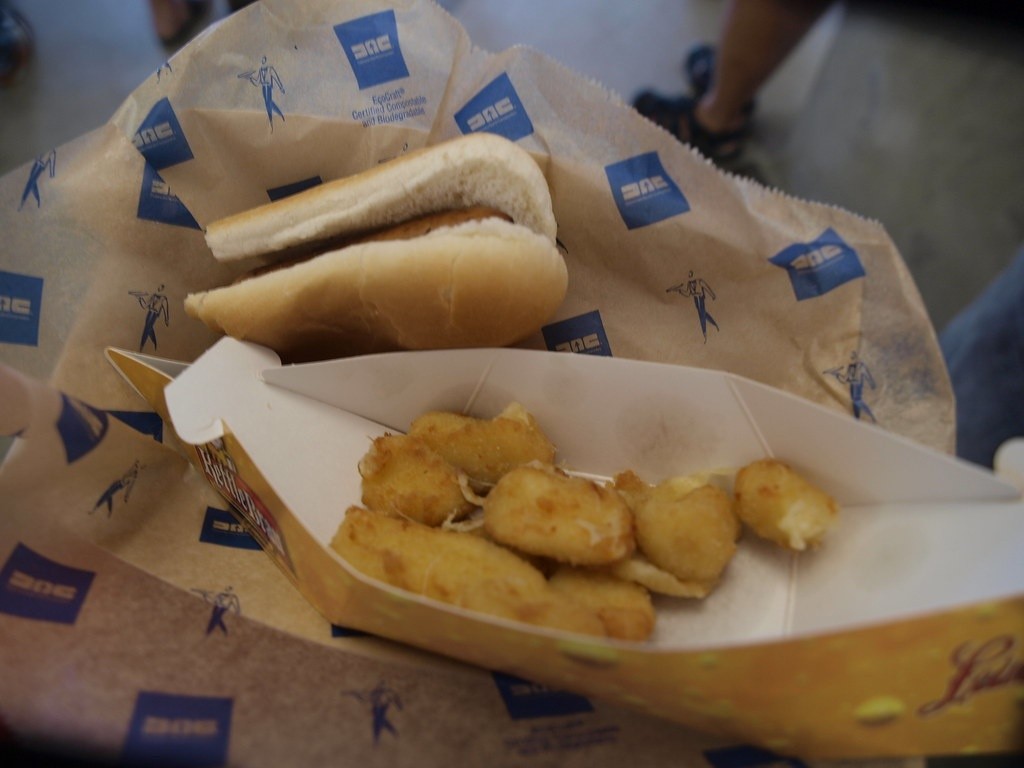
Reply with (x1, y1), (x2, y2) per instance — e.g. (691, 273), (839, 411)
(184, 133), (568, 364)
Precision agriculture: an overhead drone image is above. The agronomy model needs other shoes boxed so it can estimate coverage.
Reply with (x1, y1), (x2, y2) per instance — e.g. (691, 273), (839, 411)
(632, 88), (742, 160)
(0, 0), (35, 70)
(162, 0), (206, 45)
(686, 45), (755, 115)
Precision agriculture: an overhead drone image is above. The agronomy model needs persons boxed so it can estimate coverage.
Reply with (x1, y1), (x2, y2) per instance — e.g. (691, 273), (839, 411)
(148, 0), (254, 46)
(631, 0), (1024, 326)
